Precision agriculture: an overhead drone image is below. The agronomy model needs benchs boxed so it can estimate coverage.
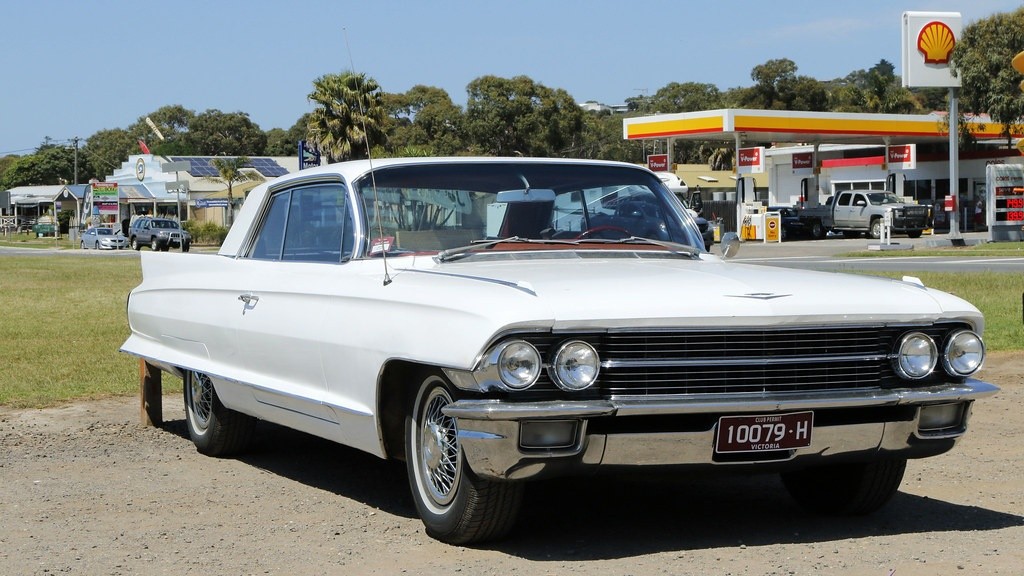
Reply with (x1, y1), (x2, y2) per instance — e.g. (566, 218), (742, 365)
(367, 228), (484, 252)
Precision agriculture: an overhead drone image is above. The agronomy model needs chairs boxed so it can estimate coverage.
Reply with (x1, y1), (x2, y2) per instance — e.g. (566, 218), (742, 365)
(497, 200), (555, 240)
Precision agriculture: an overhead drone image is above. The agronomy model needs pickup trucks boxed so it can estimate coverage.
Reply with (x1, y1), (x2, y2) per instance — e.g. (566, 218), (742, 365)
(801, 188), (932, 234)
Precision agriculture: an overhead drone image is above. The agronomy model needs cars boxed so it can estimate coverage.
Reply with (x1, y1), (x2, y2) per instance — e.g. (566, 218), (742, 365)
(80, 229), (126, 251)
(656, 190), (715, 253)
(120, 158), (996, 543)
(770, 203), (810, 238)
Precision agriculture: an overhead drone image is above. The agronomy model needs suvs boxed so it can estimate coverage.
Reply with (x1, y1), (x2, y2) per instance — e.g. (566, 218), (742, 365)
(129, 216), (192, 253)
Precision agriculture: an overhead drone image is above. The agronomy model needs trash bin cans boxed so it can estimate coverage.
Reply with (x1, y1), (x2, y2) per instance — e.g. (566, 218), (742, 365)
(708, 221), (725, 243)
